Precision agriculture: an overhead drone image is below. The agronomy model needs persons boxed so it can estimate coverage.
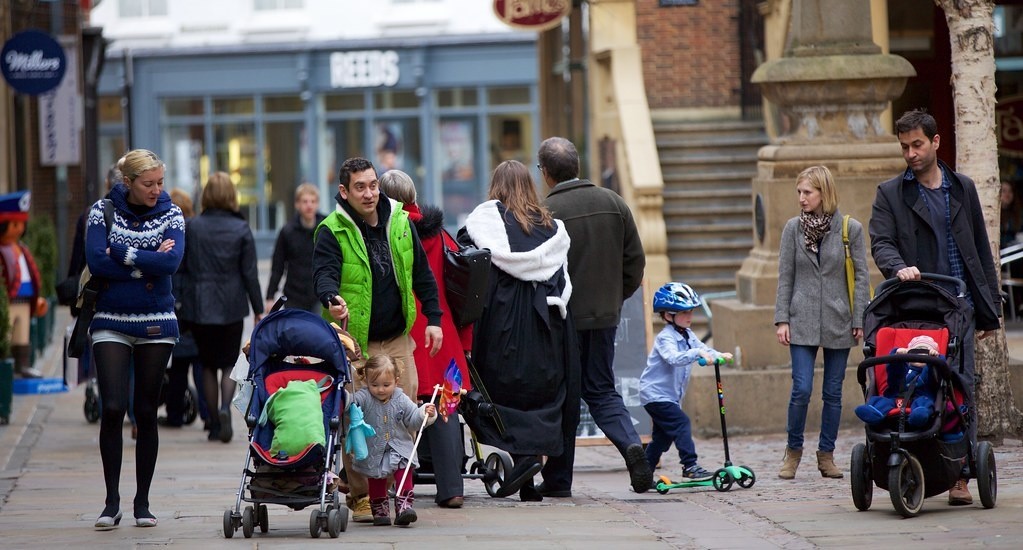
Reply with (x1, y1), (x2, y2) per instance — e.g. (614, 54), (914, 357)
(76, 109), (1023, 528)
(0, 190), (49, 380)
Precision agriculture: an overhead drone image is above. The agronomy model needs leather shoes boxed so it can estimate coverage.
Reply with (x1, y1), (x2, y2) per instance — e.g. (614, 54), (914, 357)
(949, 480), (974, 505)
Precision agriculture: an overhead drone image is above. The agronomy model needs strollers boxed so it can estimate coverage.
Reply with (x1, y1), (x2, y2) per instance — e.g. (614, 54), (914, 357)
(68, 264), (199, 426)
(411, 395), (514, 500)
(847, 272), (998, 519)
(222, 294), (350, 540)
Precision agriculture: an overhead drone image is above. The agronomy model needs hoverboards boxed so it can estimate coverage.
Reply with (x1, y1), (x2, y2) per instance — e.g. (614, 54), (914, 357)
(651, 356), (757, 494)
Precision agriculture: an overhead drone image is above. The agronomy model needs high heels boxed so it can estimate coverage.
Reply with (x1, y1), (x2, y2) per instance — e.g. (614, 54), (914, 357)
(95, 506), (123, 527)
(136, 511), (158, 527)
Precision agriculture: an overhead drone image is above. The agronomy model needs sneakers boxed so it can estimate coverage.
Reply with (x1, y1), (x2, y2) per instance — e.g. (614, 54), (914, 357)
(352, 501), (374, 522)
(628, 480), (658, 492)
(681, 465), (714, 483)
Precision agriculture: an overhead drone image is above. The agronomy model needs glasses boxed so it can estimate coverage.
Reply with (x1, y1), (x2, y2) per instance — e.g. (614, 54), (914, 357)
(536, 164), (544, 171)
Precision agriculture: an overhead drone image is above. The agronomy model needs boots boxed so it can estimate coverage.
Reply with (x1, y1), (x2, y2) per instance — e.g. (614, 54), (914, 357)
(816, 450), (844, 478)
(368, 497), (392, 525)
(779, 448), (803, 480)
(394, 490), (417, 526)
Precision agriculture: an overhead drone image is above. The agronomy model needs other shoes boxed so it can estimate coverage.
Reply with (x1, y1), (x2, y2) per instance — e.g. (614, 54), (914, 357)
(440, 496), (464, 508)
(625, 443), (653, 494)
(536, 481), (572, 498)
(496, 454), (544, 501)
(208, 407), (233, 443)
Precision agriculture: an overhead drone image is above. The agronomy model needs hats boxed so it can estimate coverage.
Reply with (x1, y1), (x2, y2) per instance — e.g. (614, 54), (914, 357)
(907, 335), (939, 355)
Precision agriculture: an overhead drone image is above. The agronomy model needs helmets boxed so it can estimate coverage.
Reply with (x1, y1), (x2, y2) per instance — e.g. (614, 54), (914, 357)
(653, 282), (703, 313)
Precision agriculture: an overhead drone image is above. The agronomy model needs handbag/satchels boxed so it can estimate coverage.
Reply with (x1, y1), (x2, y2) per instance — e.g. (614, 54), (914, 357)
(75, 264), (98, 313)
(843, 214), (874, 322)
(258, 374), (334, 458)
(439, 227), (492, 329)
